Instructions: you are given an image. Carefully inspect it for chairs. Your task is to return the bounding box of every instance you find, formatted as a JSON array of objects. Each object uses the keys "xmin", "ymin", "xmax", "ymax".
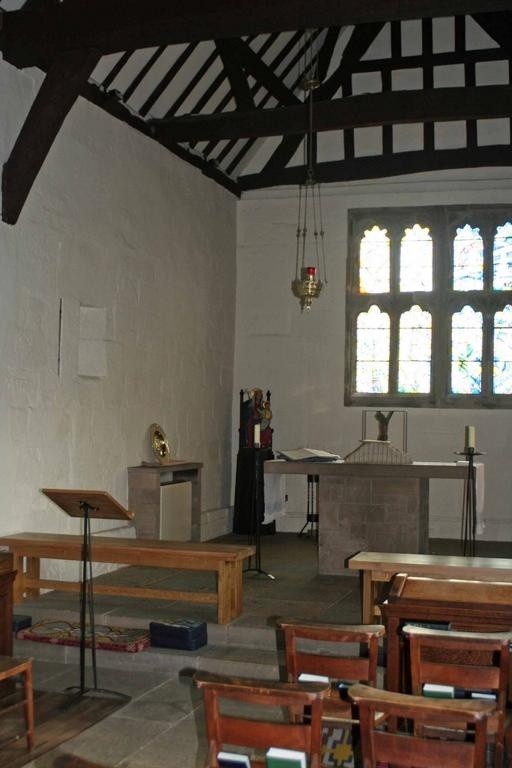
[
  {"xmin": 1, "ymin": 653, "xmax": 37, "ymax": 754},
  {"xmin": 345, "ymin": 682, "xmax": 498, "ymax": 768},
  {"xmin": 192, "ymin": 668, "xmax": 330, "ymax": 768},
  {"xmin": 265, "ymin": 615, "xmax": 386, "ymax": 730},
  {"xmin": 401, "ymin": 625, "xmax": 511, "ymax": 768}
]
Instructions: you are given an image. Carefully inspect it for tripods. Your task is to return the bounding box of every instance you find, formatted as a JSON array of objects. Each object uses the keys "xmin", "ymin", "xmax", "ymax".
[
  {"xmin": 62, "ymin": 518, "xmax": 131, "ymax": 699},
  {"xmin": 242, "ymin": 466, "xmax": 275, "ymax": 579}
]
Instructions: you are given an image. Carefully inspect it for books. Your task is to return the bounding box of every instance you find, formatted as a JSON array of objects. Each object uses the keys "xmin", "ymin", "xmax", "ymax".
[
  {"xmin": 337, "ymin": 682, "xmax": 352, "ymax": 701},
  {"xmin": 265, "ymin": 746, "xmax": 307, "ymax": 767},
  {"xmin": 295, "ymin": 673, "xmax": 330, "ymax": 685},
  {"xmin": 216, "ymin": 751, "xmax": 251, "ymax": 767},
  {"xmin": 470, "ymin": 692, "xmax": 497, "ymax": 700},
  {"xmin": 422, "ymin": 682, "xmax": 455, "ymax": 699}
]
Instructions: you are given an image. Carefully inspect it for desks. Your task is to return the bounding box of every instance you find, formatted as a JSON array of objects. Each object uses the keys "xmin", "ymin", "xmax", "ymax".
[{"xmin": 265, "ymin": 459, "xmax": 484, "ymax": 578}]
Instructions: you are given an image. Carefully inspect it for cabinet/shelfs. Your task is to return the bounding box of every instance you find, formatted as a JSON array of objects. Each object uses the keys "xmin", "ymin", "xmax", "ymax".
[
  {"xmin": 127, "ymin": 461, "xmax": 204, "ymax": 545},
  {"xmin": 376, "ymin": 572, "xmax": 510, "ymax": 732},
  {"xmin": 0, "ymin": 550, "xmax": 18, "ymax": 699}
]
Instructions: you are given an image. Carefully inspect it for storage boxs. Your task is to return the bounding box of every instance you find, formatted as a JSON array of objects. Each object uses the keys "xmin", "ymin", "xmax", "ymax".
[{"xmin": 148, "ymin": 615, "xmax": 208, "ymax": 652}]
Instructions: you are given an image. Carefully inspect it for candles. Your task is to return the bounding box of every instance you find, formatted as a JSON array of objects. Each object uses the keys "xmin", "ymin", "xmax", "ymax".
[
  {"xmin": 253, "ymin": 425, "xmax": 261, "ymax": 444},
  {"xmin": 304, "ymin": 266, "xmax": 317, "ymax": 280},
  {"xmin": 463, "ymin": 424, "xmax": 477, "ymax": 450}
]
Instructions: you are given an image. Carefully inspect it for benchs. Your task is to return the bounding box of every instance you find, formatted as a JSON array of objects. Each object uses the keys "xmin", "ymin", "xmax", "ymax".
[
  {"xmin": 348, "ymin": 550, "xmax": 511, "ymax": 625},
  {"xmin": 1, "ymin": 531, "xmax": 256, "ymax": 625}
]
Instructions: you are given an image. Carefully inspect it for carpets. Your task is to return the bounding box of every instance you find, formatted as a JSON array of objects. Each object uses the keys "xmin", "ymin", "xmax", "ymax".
[{"xmin": 0, "ymin": 687, "xmax": 126, "ymax": 768}]
